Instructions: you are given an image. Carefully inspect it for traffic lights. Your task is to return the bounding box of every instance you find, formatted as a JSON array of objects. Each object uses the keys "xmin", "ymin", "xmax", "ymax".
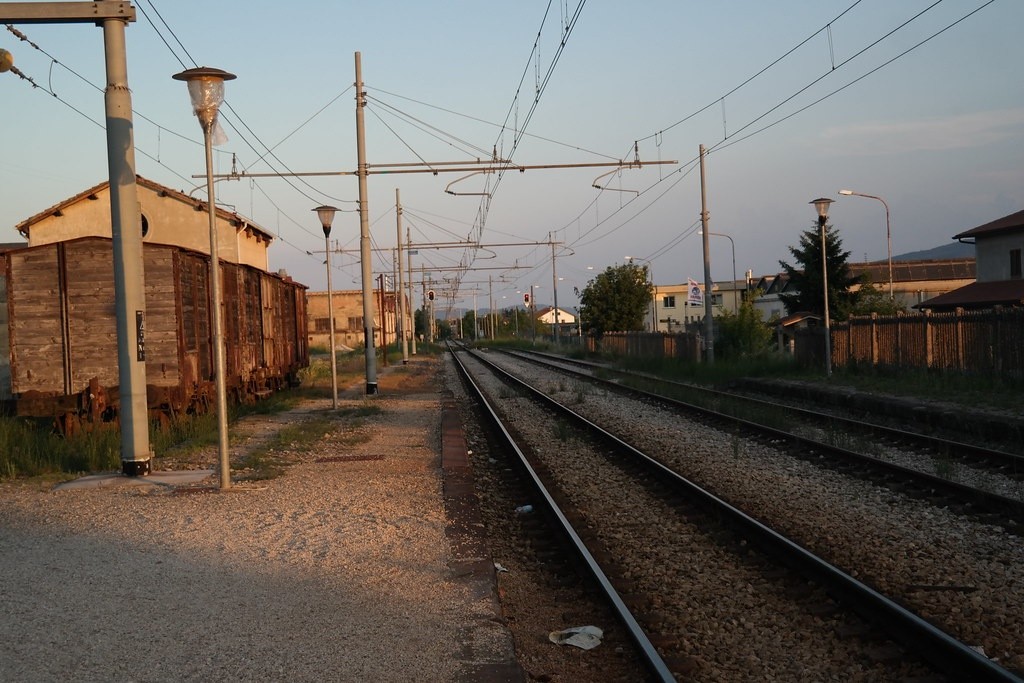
[
  {"xmin": 525, "ymin": 294, "xmax": 529, "ymax": 303},
  {"xmin": 428, "ymin": 291, "xmax": 434, "ymax": 301}
]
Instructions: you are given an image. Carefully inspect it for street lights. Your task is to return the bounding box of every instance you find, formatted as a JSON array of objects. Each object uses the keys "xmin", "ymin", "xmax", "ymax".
[
  {"xmin": 536, "ymin": 286, "xmax": 554, "ymax": 339},
  {"xmin": 697, "ymin": 230, "xmax": 738, "ymax": 359},
  {"xmin": 502, "ymin": 296, "xmax": 519, "ymax": 342},
  {"xmin": 559, "ymin": 278, "xmax": 582, "ymax": 345},
  {"xmin": 516, "ymin": 291, "xmax": 533, "ymax": 340},
  {"xmin": 839, "ymin": 191, "xmax": 894, "ymax": 311},
  {"xmin": 810, "ymin": 196, "xmax": 835, "ymax": 375},
  {"xmin": 587, "ymin": 267, "xmax": 607, "ymax": 288},
  {"xmin": 311, "ymin": 207, "xmax": 343, "ymax": 408},
  {"xmin": 175, "ymin": 67, "xmax": 238, "ymax": 487},
  {"xmin": 624, "ymin": 256, "xmax": 655, "ymax": 332}
]
